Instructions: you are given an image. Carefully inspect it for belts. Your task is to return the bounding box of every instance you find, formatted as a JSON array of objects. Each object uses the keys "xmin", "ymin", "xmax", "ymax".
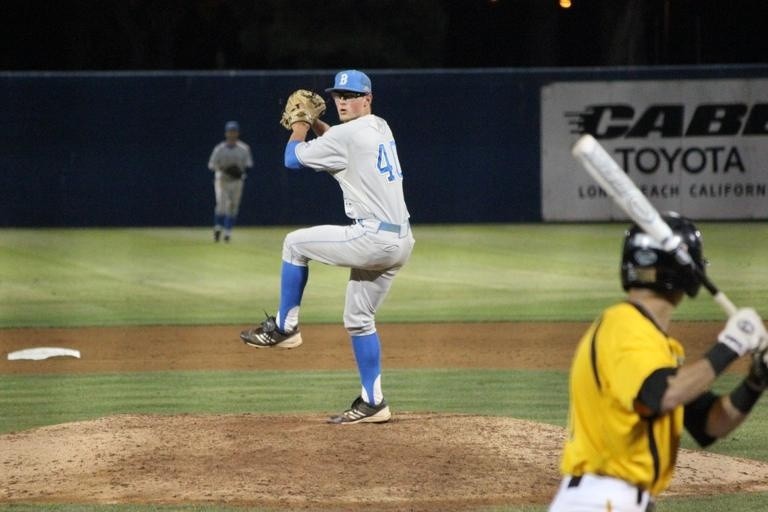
[
  {"xmin": 569, "ymin": 474, "xmax": 656, "ymax": 512},
  {"xmin": 379, "ymin": 222, "xmax": 400, "ymax": 234}
]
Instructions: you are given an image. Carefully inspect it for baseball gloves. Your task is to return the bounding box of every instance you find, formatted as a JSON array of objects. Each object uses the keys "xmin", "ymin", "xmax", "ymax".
[
  {"xmin": 224, "ymin": 165, "xmax": 243, "ymax": 180},
  {"xmin": 282, "ymin": 89, "xmax": 329, "ymax": 129}
]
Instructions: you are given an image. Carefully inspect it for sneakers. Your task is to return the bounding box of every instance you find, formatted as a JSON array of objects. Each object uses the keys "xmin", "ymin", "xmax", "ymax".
[
  {"xmin": 240, "ymin": 315, "xmax": 303, "ymax": 347},
  {"xmin": 327, "ymin": 396, "xmax": 391, "ymax": 425},
  {"xmin": 212, "ymin": 232, "xmax": 230, "ymax": 241}
]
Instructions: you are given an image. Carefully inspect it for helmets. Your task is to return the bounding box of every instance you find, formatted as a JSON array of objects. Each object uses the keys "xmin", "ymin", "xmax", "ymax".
[{"xmin": 620, "ymin": 211, "xmax": 703, "ymax": 303}]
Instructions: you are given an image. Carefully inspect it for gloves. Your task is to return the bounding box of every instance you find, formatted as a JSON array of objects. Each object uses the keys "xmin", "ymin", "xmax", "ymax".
[{"xmin": 717, "ymin": 308, "xmax": 767, "ymax": 388}]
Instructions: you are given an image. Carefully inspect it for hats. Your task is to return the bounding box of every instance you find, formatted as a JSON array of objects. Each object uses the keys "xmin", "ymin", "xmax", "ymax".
[
  {"xmin": 326, "ymin": 70, "xmax": 371, "ymax": 92},
  {"xmin": 227, "ymin": 122, "xmax": 238, "ymax": 130}
]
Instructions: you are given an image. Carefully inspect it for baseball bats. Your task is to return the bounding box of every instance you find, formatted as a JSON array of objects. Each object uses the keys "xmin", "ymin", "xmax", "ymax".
[{"xmin": 571, "ymin": 133, "xmax": 762, "ymax": 347}]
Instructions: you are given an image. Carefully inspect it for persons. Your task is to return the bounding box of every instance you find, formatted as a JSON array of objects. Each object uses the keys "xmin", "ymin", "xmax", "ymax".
[
  {"xmin": 240, "ymin": 70, "xmax": 417, "ymax": 424},
  {"xmin": 547, "ymin": 202, "xmax": 768, "ymax": 512},
  {"xmin": 207, "ymin": 121, "xmax": 255, "ymax": 243}
]
